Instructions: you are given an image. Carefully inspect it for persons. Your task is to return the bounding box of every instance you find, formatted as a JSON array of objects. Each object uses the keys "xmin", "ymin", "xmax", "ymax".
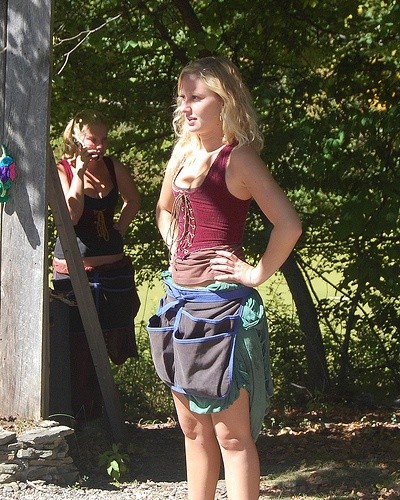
[
  {"xmin": 147, "ymin": 57, "xmax": 302, "ymax": 500},
  {"xmin": 53, "ymin": 109, "xmax": 142, "ymax": 421}
]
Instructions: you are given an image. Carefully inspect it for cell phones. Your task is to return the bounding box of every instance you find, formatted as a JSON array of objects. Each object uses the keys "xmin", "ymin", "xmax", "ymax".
[{"xmin": 71, "ymin": 134, "xmax": 98, "ymax": 161}]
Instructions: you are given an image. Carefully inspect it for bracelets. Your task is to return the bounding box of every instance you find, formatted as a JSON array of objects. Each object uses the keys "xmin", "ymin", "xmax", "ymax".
[{"xmin": 250, "ymin": 267, "xmax": 256, "ymax": 286}]
